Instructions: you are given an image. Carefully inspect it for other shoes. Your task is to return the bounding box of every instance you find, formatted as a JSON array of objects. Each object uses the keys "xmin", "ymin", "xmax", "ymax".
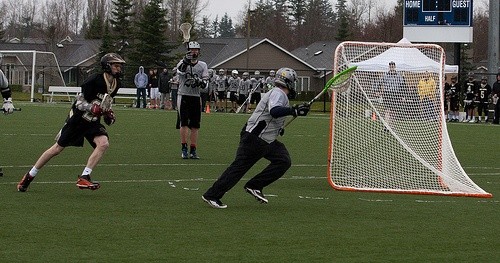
[
  {"xmin": 449, "ymin": 118, "xmax": 489, "ymax": 123},
  {"xmin": 189, "ymin": 151, "xmax": 200, "ymax": 159},
  {"xmin": 181, "ymin": 149, "xmax": 189, "ymax": 158}
]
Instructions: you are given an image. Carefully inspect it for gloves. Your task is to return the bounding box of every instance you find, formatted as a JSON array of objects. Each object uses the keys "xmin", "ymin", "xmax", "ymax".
[
  {"xmin": 104, "ymin": 111, "xmax": 116, "ymax": 126},
  {"xmin": 2, "ymin": 97, "xmax": 15, "ymax": 115},
  {"xmin": 182, "ymin": 50, "xmax": 197, "ymax": 64},
  {"xmin": 190, "ymin": 76, "xmax": 203, "ymax": 87},
  {"xmin": 291, "ymin": 102, "xmax": 310, "ymax": 118},
  {"xmin": 89, "ymin": 102, "xmax": 102, "ymax": 116}
]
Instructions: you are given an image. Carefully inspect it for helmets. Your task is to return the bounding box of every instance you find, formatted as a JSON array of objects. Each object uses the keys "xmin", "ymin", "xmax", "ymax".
[
  {"xmin": 274, "ymin": 67, "xmax": 299, "ymax": 97},
  {"xmin": 101, "ymin": 53, "xmax": 126, "ymax": 81},
  {"xmin": 242, "ymin": 72, "xmax": 249, "ymax": 79},
  {"xmin": 467, "ymin": 72, "xmax": 474, "ymax": 79},
  {"xmin": 232, "ymin": 70, "xmax": 238, "ymax": 79},
  {"xmin": 270, "ymin": 70, "xmax": 276, "ymax": 76},
  {"xmin": 219, "ymin": 69, "xmax": 224, "ymax": 74},
  {"xmin": 187, "ymin": 41, "xmax": 200, "ymax": 49},
  {"xmin": 451, "ymin": 75, "xmax": 457, "ymax": 81}
]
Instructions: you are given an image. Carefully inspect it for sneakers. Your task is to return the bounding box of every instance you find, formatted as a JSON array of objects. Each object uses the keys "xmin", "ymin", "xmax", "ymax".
[
  {"xmin": 16, "ymin": 171, "xmax": 36, "ymax": 192},
  {"xmin": 201, "ymin": 195, "xmax": 228, "ymax": 209},
  {"xmin": 243, "ymin": 185, "xmax": 268, "ymax": 205},
  {"xmin": 75, "ymin": 174, "xmax": 101, "ymax": 191}
]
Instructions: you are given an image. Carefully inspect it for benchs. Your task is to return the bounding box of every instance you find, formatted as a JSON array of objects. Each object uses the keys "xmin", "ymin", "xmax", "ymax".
[{"xmin": 42, "ymin": 86, "xmax": 159, "ymax": 108}]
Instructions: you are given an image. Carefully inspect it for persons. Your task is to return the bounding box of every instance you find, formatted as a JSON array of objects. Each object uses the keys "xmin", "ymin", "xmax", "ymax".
[
  {"xmin": 134, "ymin": 65, "xmax": 276, "ymax": 129},
  {"xmin": 409, "ymin": 70, "xmax": 500, "ymax": 123},
  {"xmin": 177, "ymin": 41, "xmax": 209, "ymax": 159},
  {"xmin": 376, "ymin": 61, "xmax": 405, "ymax": 132},
  {"xmin": 17, "ymin": 52, "xmax": 126, "ymax": 192},
  {"xmin": 201, "ymin": 67, "xmax": 310, "ymax": 209},
  {"xmin": 0, "ymin": 69, "xmax": 14, "ymax": 176}
]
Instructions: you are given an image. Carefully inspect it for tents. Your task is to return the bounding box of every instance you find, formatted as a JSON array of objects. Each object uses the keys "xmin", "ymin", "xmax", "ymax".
[{"xmin": 346, "ymin": 36, "xmax": 458, "ymax": 117}]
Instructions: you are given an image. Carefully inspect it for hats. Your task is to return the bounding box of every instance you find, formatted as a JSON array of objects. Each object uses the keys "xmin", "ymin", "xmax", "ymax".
[
  {"xmin": 481, "ymin": 76, "xmax": 488, "ymax": 80},
  {"xmin": 254, "ymin": 71, "xmax": 260, "ymax": 75}
]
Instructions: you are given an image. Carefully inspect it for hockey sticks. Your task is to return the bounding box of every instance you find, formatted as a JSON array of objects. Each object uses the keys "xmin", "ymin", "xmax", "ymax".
[
  {"xmin": 99, "ymin": 93, "xmax": 113, "ymax": 118},
  {"xmin": 0, "ymin": 108, "xmax": 21, "ymax": 111},
  {"xmin": 281, "ymin": 65, "xmax": 358, "ymax": 130},
  {"xmin": 236, "ymin": 78, "xmax": 264, "ymax": 114},
  {"xmin": 180, "ymin": 22, "xmax": 193, "ymax": 77}
]
